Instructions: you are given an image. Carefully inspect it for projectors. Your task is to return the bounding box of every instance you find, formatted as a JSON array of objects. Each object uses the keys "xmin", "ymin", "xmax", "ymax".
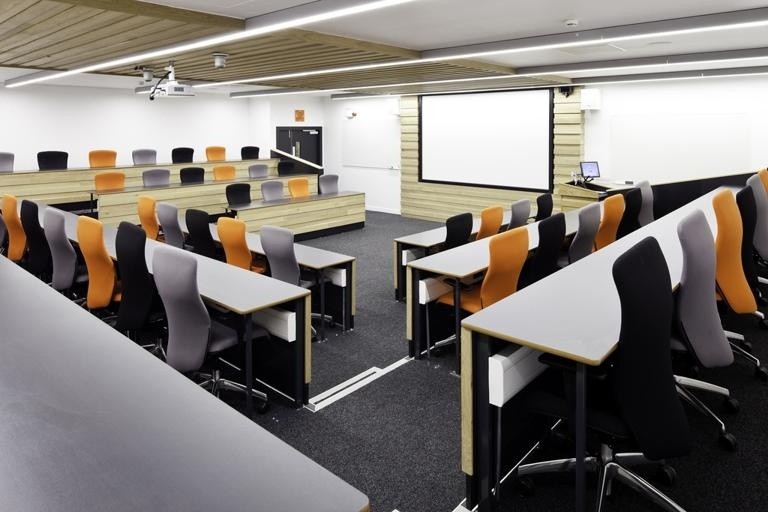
[{"xmin": 134, "ymin": 81, "xmax": 195, "ymax": 101}]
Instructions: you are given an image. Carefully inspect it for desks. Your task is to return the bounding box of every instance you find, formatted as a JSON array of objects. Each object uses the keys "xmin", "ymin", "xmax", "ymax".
[
  {"xmin": 0, "ymin": 256, "xmax": 370, "ymax": 512},
  {"xmin": 394, "ymin": 205, "xmax": 539, "ymax": 301}
]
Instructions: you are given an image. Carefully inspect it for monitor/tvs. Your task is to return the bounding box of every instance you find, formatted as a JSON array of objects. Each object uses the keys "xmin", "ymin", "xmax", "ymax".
[{"xmin": 580, "ymin": 161, "xmax": 601, "ymax": 178}]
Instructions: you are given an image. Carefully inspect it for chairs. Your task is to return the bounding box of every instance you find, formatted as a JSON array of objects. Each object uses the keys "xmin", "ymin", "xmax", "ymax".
[
  {"xmin": 89, "ymin": 149, "xmax": 117, "ymax": 168},
  {"xmin": 37, "ymin": 151, "xmax": 68, "ymax": 170},
  {"xmin": 172, "ymin": 148, "xmax": 194, "ymax": 163},
  {"xmin": 0, "ymin": 152, "xmax": 14, "ymax": 172},
  {"xmin": 241, "ymin": 146, "xmax": 259, "ymax": 160},
  {"xmin": 206, "ymin": 146, "xmax": 226, "ymax": 161},
  {"xmin": 132, "ymin": 149, "xmax": 157, "ymax": 165}
]
